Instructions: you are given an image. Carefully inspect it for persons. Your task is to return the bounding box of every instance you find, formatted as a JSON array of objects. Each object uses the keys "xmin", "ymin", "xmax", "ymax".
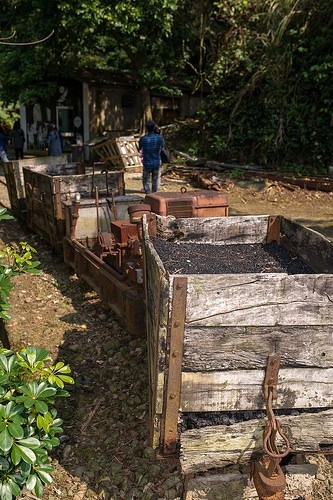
[
  {"xmin": 44, "ymin": 124, "xmax": 61, "ymax": 156},
  {"xmin": 136, "ymin": 120, "xmax": 165, "ymax": 194},
  {"xmin": 10, "ymin": 121, "xmax": 26, "ymax": 161},
  {"xmin": 0, "ymin": 120, "xmax": 11, "ymax": 160}
]
